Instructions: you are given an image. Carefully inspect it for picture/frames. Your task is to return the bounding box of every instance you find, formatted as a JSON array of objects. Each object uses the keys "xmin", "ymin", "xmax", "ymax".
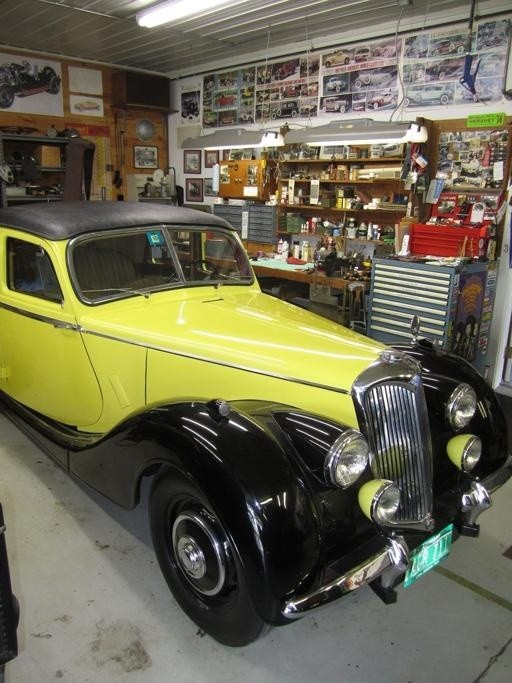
[
  {"xmin": 183, "ymin": 148, "xmax": 254, "ymax": 202},
  {"xmin": 133, "ymin": 144, "xmax": 159, "ymax": 170}
]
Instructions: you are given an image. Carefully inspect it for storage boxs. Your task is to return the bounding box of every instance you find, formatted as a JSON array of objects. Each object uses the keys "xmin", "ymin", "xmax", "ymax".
[{"xmin": 31, "ymin": 145, "xmax": 62, "ymax": 170}]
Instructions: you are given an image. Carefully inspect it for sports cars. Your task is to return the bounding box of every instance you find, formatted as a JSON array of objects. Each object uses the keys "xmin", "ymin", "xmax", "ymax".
[{"xmin": 1, "ymin": 202, "xmax": 510, "ymax": 648}]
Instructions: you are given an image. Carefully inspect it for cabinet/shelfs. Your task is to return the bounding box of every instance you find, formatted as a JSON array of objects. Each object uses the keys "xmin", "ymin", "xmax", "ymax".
[
  {"xmin": 1, "ymin": 133, "xmax": 86, "ymax": 209},
  {"xmin": 276, "ymin": 158, "xmax": 406, "ymax": 246}
]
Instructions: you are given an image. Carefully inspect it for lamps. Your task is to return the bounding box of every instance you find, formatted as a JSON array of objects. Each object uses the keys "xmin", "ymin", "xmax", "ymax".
[
  {"xmin": 134, "ymin": 1, "xmax": 227, "ymax": 29},
  {"xmin": 179, "ymin": 4, "xmax": 428, "ymax": 150}
]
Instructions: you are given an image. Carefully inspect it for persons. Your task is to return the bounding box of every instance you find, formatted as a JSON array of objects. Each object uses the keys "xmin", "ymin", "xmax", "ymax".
[{"xmin": 453, "ymin": 314, "xmax": 479, "ymax": 361}]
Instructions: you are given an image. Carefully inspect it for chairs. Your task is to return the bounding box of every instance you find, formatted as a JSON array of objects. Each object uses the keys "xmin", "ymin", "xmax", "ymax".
[{"xmin": 29, "ymin": 242, "xmax": 162, "ymax": 306}]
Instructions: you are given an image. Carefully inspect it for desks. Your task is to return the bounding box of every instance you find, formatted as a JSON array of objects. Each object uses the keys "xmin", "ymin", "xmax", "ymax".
[{"xmin": 172, "ymin": 254, "xmax": 368, "ymax": 320}]
